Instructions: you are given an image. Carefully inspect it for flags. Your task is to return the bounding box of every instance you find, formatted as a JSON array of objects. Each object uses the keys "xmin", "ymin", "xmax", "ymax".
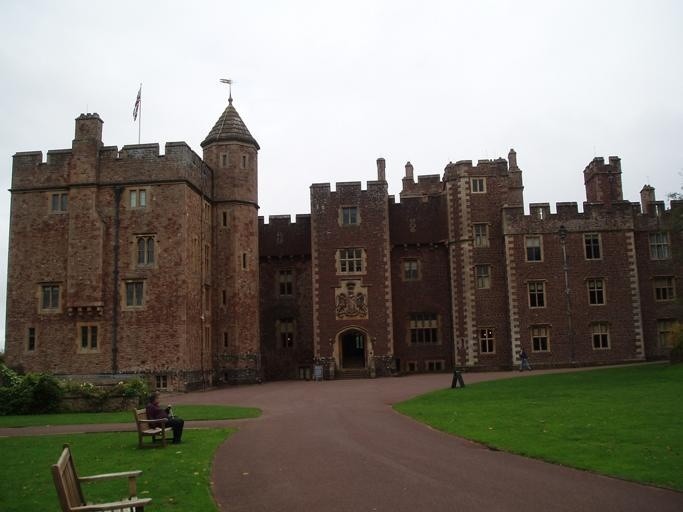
[{"xmin": 132, "ymin": 89, "xmax": 141, "ymax": 121}]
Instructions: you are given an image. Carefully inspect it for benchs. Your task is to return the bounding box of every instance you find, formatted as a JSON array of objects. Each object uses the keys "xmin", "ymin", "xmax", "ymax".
[
  {"xmin": 133, "ymin": 407, "xmax": 175, "ymax": 448},
  {"xmin": 52, "ymin": 444, "xmax": 153, "ymax": 511}
]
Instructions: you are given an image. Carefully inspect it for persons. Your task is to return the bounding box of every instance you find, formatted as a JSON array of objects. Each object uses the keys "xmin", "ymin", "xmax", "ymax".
[
  {"xmin": 518, "ymin": 348, "xmax": 532, "ymax": 371},
  {"xmin": 146, "ymin": 393, "xmax": 184, "ymax": 444}
]
